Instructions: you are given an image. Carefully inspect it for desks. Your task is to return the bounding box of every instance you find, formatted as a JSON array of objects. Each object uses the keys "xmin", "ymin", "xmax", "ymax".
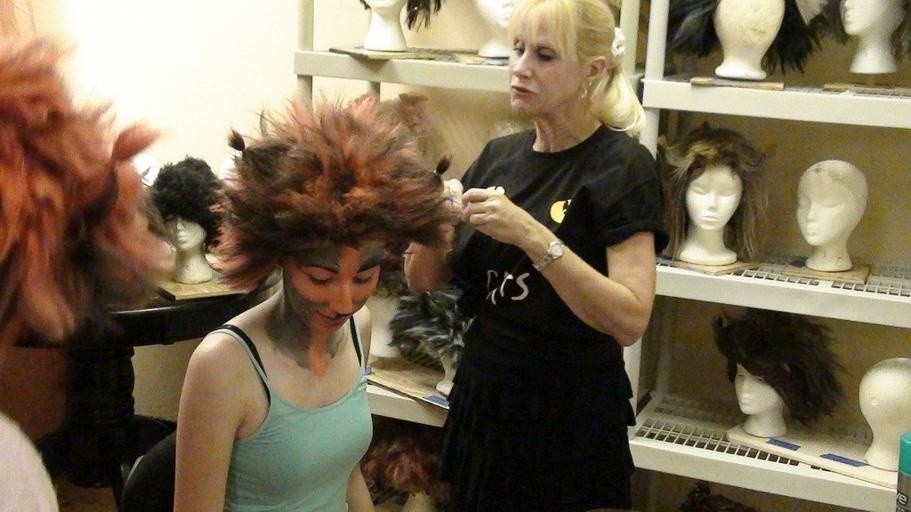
[{"xmin": 8, "ymin": 268, "xmax": 268, "ymax": 489}]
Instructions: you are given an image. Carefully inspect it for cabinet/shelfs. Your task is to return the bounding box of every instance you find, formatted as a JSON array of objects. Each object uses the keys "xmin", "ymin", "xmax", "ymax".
[
  {"xmin": 293, "ymin": 1, "xmax": 641, "ymax": 428},
  {"xmin": 627, "ymin": 1, "xmax": 911, "ymax": 512}
]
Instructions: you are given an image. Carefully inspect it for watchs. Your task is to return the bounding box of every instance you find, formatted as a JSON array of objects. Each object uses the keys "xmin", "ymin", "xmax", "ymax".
[{"xmin": 531, "ymin": 239, "xmax": 566, "ymax": 273}]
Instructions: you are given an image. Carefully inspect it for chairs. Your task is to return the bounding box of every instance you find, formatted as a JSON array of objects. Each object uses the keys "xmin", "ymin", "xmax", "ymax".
[{"xmin": 121, "ymin": 432, "xmax": 177, "ymax": 511}]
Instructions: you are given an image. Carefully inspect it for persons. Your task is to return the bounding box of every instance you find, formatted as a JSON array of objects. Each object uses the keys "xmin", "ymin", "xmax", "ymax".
[
  {"xmin": 665, "ymin": 0, "xmax": 824, "ymax": 82},
  {"xmin": 711, "ymin": 306, "xmax": 845, "ymax": 437},
  {"xmin": 405, "ymin": 2, "xmax": 670, "ymax": 511},
  {"xmin": 795, "ymin": 161, "xmax": 873, "ymax": 272},
  {"xmin": 858, "ymin": 355, "xmax": 910, "ymax": 468},
  {"xmin": 810, "ymin": 1, "xmax": 910, "ymax": 78},
  {"xmin": 1, "ymin": 0, "xmax": 168, "ymax": 509},
  {"xmin": 170, "ymin": 95, "xmax": 463, "ymax": 510},
  {"xmin": 359, "ymin": 0, "xmax": 443, "ymax": 56},
  {"xmin": 147, "ymin": 156, "xmax": 230, "ymax": 285},
  {"xmin": 469, "ymin": 0, "xmax": 527, "ymax": 61},
  {"xmin": 658, "ymin": 116, "xmax": 773, "ymax": 266}
]
